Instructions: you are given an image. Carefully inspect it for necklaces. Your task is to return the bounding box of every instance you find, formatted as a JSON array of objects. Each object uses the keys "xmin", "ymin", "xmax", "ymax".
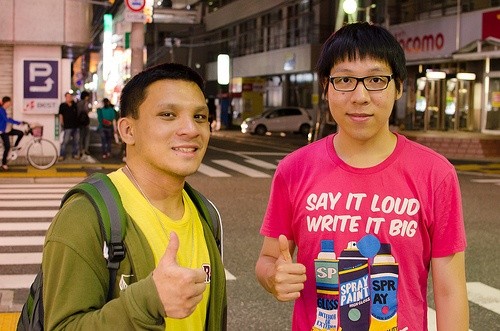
[{"xmin": 126, "ymin": 164, "xmax": 195, "ymax": 268}]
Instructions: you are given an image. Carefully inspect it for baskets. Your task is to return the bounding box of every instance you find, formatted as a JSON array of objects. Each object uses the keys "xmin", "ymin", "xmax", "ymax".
[{"xmin": 32, "ymin": 126, "xmax": 43, "ymax": 137}]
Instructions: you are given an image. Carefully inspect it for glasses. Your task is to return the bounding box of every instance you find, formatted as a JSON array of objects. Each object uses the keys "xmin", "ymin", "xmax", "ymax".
[{"xmin": 328, "ymin": 72, "xmax": 401, "ymax": 92}]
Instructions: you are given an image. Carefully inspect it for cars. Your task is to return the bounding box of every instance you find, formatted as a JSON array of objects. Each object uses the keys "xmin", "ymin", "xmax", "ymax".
[{"xmin": 241, "ymin": 106, "xmax": 313, "ymax": 138}]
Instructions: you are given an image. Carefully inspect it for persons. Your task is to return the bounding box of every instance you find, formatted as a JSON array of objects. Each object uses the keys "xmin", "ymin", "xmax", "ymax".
[
  {"xmin": 0, "ymin": 96, "xmax": 27, "ymax": 171},
  {"xmin": 43, "ymin": 63, "xmax": 227, "ymax": 331},
  {"xmin": 255, "ymin": 23, "xmax": 469, "ymax": 331},
  {"xmin": 57, "ymin": 91, "xmax": 127, "ymax": 162}
]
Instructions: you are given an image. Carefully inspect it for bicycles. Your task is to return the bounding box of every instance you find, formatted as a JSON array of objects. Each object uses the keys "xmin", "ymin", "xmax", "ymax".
[{"xmin": 0, "ymin": 122, "xmax": 58, "ymax": 171}]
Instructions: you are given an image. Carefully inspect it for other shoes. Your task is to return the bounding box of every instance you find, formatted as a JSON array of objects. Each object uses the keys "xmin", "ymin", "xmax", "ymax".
[
  {"xmin": 2, "ymin": 164, "xmax": 8, "ymax": 169},
  {"xmin": 16, "ymin": 145, "xmax": 21, "ymax": 148},
  {"xmin": 57, "ymin": 155, "xmax": 63, "ymax": 161},
  {"xmin": 72, "ymin": 154, "xmax": 78, "ymax": 159}
]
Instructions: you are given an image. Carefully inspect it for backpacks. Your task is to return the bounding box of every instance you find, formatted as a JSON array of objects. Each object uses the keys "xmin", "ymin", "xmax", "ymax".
[{"xmin": 16, "ymin": 172, "xmax": 222, "ymax": 331}]
